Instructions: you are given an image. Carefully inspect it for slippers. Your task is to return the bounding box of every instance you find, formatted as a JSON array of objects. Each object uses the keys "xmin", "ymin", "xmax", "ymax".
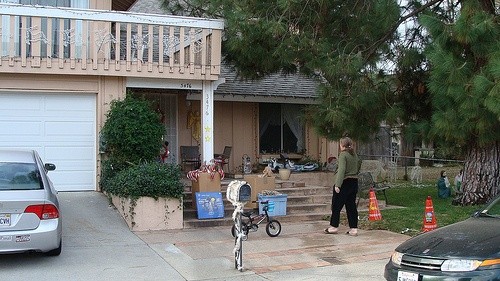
[
  {"xmin": 323, "ymin": 228, "xmax": 338, "ymax": 235},
  {"xmin": 346, "ymin": 229, "xmax": 357, "ymax": 237}
]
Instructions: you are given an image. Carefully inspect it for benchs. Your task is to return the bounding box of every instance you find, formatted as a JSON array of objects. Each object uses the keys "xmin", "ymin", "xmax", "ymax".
[{"xmin": 356, "ymin": 172, "xmax": 390, "ymax": 207}]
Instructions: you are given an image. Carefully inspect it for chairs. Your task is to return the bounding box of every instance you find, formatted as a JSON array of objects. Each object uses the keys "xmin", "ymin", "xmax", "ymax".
[
  {"xmin": 214, "ymin": 146, "xmax": 232, "ymax": 173},
  {"xmin": 180, "ymin": 146, "xmax": 201, "ymax": 172}
]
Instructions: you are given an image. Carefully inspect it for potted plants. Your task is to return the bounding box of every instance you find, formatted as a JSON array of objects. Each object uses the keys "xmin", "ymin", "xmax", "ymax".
[{"xmin": 276, "ymin": 154, "xmax": 291, "ymax": 180}]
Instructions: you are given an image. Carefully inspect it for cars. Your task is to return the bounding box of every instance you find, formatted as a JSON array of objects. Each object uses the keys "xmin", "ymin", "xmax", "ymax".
[
  {"xmin": 384, "ymin": 193, "xmax": 500, "ymax": 281},
  {"xmin": 0, "ymin": 149, "xmax": 63, "ymax": 256}
]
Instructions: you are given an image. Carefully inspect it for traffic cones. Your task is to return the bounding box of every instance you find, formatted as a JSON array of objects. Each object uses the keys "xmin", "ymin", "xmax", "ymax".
[
  {"xmin": 369, "ymin": 188, "xmax": 381, "ymax": 222},
  {"xmin": 422, "ymin": 196, "xmax": 440, "ymax": 233}
]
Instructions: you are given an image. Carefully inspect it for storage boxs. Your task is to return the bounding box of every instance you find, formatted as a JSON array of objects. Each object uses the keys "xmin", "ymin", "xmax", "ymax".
[
  {"xmin": 257, "ymin": 194, "xmax": 288, "ymax": 216},
  {"xmin": 243, "ymin": 174, "xmax": 276, "ymax": 208},
  {"xmin": 192, "ymin": 172, "xmax": 221, "ymax": 208}
]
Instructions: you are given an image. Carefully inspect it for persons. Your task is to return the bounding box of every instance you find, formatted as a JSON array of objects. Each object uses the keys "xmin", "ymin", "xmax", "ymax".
[
  {"xmin": 438, "ymin": 169, "xmax": 464, "ymax": 200},
  {"xmin": 323, "ymin": 136, "xmax": 362, "ymax": 237}
]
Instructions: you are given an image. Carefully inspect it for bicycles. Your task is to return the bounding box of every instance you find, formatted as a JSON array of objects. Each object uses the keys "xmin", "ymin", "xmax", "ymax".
[{"xmin": 230, "ymin": 199, "xmax": 282, "ymax": 241}]
